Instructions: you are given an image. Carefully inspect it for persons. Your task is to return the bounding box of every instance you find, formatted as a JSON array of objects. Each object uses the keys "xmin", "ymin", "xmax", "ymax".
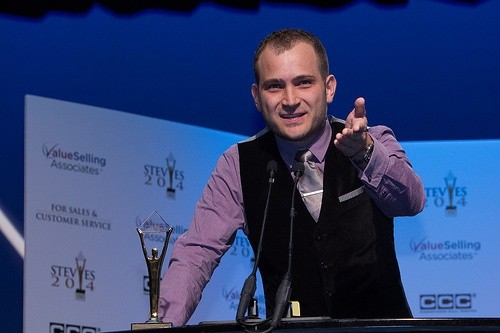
[
  {"xmin": 135, "ymin": 225, "xmax": 174, "ymax": 324},
  {"xmin": 150, "ymin": 28, "xmax": 427, "ymax": 326}
]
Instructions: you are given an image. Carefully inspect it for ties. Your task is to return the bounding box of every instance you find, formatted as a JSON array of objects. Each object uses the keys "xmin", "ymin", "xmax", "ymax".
[{"xmin": 297, "ymin": 149, "xmax": 323, "ymax": 223}]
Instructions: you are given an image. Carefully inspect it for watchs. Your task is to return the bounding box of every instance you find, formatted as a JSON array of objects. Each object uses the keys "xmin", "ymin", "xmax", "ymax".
[{"xmin": 350, "ymin": 143, "xmax": 376, "ymax": 163}]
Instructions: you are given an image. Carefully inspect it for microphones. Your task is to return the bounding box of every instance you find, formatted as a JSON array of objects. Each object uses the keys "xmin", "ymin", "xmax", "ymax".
[
  {"xmin": 271, "ymin": 161, "xmax": 305, "ymax": 328},
  {"xmin": 236, "ymin": 161, "xmax": 278, "ymax": 322}
]
